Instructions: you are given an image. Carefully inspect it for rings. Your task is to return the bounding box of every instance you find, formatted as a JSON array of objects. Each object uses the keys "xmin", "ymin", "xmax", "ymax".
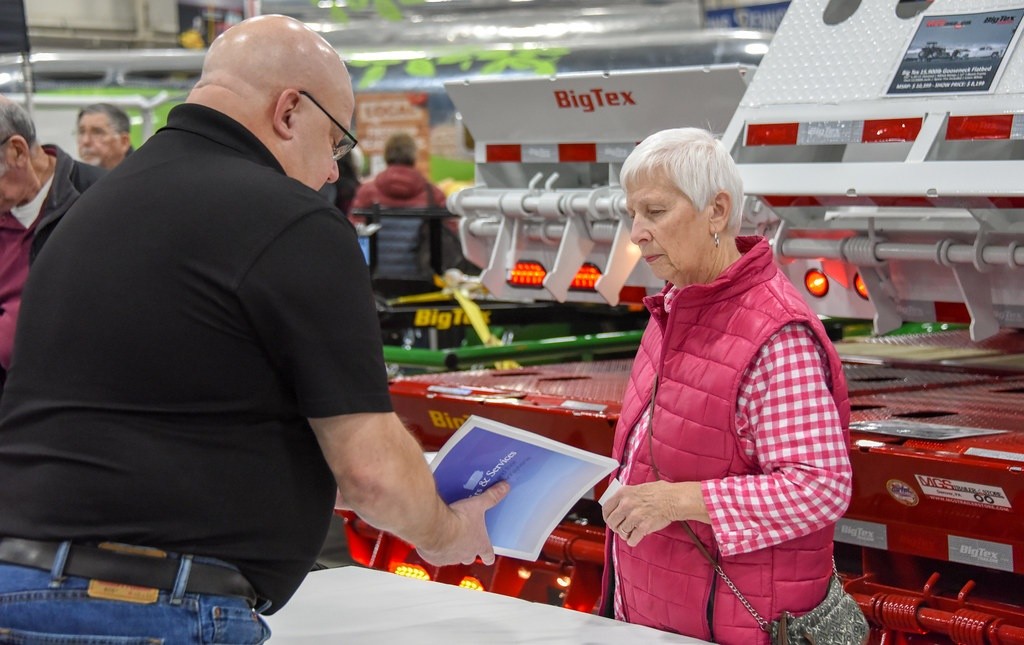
[{"xmin": 620, "ymin": 526, "xmax": 629, "ymax": 535}]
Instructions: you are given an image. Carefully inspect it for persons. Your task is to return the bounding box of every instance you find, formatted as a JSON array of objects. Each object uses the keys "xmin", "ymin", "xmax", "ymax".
[
  {"xmin": 598, "ymin": 125, "xmax": 853, "ymax": 644},
  {"xmin": 1, "ymin": 13, "xmax": 510, "ymax": 645}
]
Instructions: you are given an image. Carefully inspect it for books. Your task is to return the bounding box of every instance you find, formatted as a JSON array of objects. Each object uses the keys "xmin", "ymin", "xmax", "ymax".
[{"xmin": 423, "ymin": 414, "xmax": 620, "ymax": 562}]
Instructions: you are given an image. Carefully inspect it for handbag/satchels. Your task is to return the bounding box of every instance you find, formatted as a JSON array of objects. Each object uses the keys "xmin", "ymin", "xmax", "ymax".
[{"xmin": 764, "ymin": 577, "xmax": 870, "ymax": 645}]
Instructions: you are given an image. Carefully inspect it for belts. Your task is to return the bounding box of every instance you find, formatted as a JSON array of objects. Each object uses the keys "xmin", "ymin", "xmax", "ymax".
[{"xmin": 0, "ymin": 536, "xmax": 259, "ymax": 606}]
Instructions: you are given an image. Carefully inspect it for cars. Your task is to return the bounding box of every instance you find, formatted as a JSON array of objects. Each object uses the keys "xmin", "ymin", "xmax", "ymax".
[{"xmin": 949, "ymin": 43, "xmax": 1002, "ymax": 61}]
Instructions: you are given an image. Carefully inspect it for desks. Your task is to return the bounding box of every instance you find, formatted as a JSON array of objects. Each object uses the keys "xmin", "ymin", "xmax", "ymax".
[{"xmin": 253, "ymin": 566, "xmax": 718, "ymax": 645}]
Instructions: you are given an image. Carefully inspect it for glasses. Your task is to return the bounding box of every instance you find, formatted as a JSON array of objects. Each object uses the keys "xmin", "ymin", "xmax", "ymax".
[{"xmin": 298, "ymin": 90, "xmax": 357, "ymax": 161}]
